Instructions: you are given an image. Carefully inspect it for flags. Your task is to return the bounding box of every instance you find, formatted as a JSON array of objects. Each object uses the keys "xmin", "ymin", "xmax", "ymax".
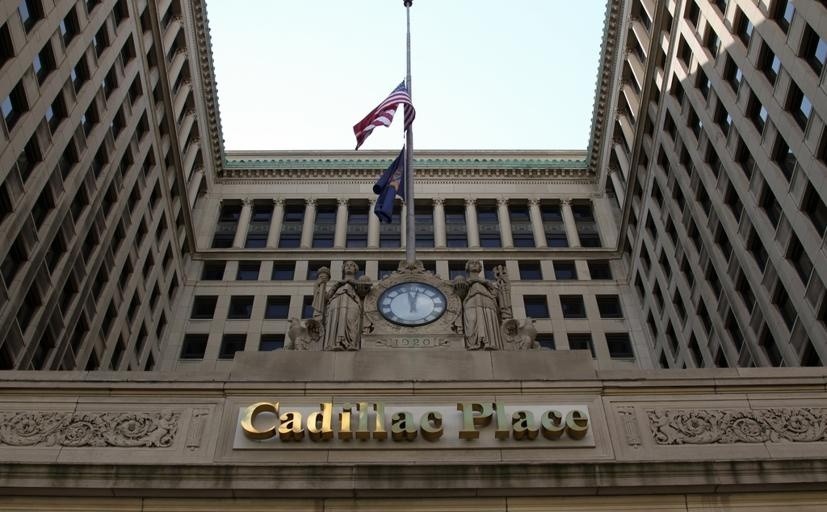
[
  {"xmin": 373, "ymin": 144, "xmax": 405, "ymax": 224},
  {"xmin": 353, "ymin": 81, "xmax": 416, "ymax": 151}
]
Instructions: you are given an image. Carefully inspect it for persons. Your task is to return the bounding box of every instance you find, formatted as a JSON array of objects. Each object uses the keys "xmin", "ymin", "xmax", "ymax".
[
  {"xmin": 312, "ymin": 261, "xmax": 370, "ymax": 351},
  {"xmin": 453, "ymin": 257, "xmax": 507, "ymax": 351}
]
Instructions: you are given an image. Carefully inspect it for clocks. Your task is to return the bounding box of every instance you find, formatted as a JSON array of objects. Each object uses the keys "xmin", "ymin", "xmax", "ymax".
[{"xmin": 376, "ymin": 282, "xmax": 447, "ymax": 326}]
